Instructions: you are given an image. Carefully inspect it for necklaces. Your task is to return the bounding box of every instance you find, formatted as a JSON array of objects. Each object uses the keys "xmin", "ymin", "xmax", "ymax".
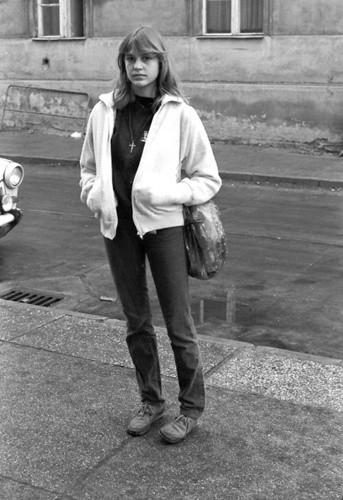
[{"xmin": 124, "ymin": 100, "xmax": 154, "ymax": 152}]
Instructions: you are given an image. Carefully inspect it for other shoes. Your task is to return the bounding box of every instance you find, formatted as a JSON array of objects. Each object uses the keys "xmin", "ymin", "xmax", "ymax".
[
  {"xmin": 128, "ymin": 403, "xmax": 165, "ymax": 435},
  {"xmin": 158, "ymin": 415, "xmax": 204, "ymax": 444}
]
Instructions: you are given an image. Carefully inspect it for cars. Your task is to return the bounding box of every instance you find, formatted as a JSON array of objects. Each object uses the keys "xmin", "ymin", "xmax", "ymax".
[{"xmin": 0, "ymin": 156, "xmax": 25, "ymax": 241}]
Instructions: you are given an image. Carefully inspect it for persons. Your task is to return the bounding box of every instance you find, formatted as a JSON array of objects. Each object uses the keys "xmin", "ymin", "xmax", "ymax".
[{"xmin": 78, "ymin": 27, "xmax": 222, "ymax": 443}]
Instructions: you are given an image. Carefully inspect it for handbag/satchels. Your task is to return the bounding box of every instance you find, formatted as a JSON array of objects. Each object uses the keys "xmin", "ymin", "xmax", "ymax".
[{"xmin": 184, "ymin": 201, "xmax": 228, "ymax": 281}]
